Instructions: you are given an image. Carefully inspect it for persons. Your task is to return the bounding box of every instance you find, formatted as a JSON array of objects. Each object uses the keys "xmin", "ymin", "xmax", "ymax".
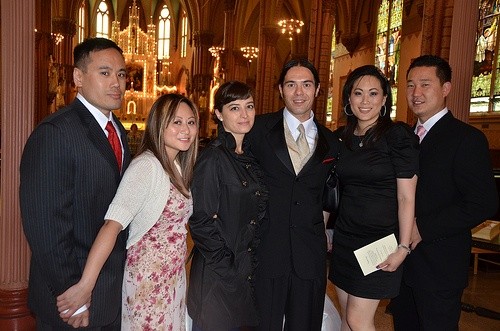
[
  {"xmin": 244, "ymin": 60, "xmax": 340, "ymax": 331},
  {"xmin": 329, "ymin": 65, "xmax": 419, "ymax": 331},
  {"xmin": 56, "ymin": 94, "xmax": 199, "ymax": 331},
  {"xmin": 390, "ymin": 55, "xmax": 494, "ymax": 331},
  {"xmin": 19, "ymin": 38, "xmax": 132, "ymax": 331},
  {"xmin": 187, "ymin": 80, "xmax": 270, "ymax": 331}
]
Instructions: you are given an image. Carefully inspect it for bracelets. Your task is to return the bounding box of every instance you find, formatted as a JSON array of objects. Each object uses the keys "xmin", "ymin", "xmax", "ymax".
[{"xmin": 398, "ymin": 244, "xmax": 411, "ymax": 254}]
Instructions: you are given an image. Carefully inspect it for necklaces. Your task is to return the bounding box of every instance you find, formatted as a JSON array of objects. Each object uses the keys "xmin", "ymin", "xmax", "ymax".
[{"xmin": 359, "ymin": 133, "xmax": 365, "ymax": 147}]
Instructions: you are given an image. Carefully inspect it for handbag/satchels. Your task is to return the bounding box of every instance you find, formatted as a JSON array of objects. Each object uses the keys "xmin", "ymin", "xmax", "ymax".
[{"xmin": 321, "ymin": 164, "xmax": 341, "ymax": 214}]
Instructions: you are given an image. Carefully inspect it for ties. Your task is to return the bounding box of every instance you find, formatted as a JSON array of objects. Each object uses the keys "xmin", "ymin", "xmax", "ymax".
[
  {"xmin": 416, "ymin": 125, "xmax": 426, "ymax": 141},
  {"xmin": 105, "ymin": 120, "xmax": 122, "ymax": 172},
  {"xmin": 296, "ymin": 124, "xmax": 310, "ymax": 161}
]
